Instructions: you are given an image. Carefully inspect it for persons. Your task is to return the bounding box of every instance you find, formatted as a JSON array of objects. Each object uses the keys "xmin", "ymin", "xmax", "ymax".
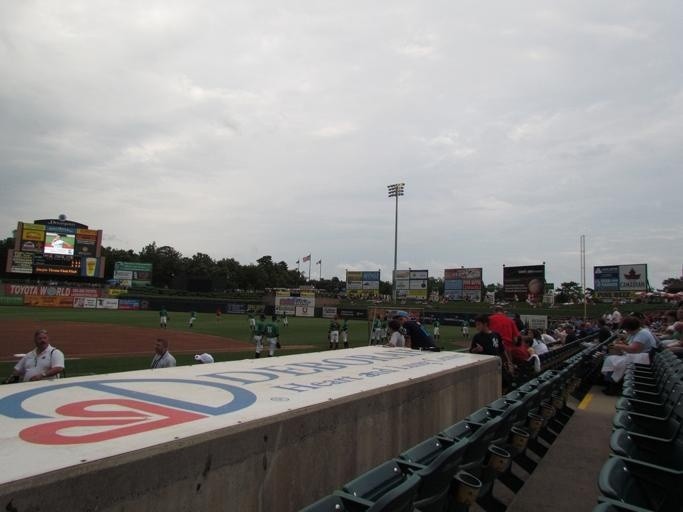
[
  {"xmin": 51, "ymin": 236, "xmax": 70, "ymax": 249},
  {"xmin": 188, "ymin": 308, "xmax": 196, "ymax": 329},
  {"xmin": 2, "ymin": 327, "xmax": 65, "ymax": 383},
  {"xmin": 193, "ymin": 352, "xmax": 214, "ymax": 364},
  {"xmin": 159, "ymin": 306, "xmax": 168, "ymax": 329},
  {"xmin": 215, "ymin": 307, "xmax": 221, "ymax": 325},
  {"xmin": 151, "ymin": 338, "xmax": 175, "ymax": 368}
]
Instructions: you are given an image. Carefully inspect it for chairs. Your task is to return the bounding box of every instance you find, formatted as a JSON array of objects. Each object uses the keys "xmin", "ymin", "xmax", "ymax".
[
  {"xmin": 398, "ymin": 416, "xmax": 504, "ymax": 472},
  {"xmin": 590, "ymin": 494, "xmax": 650, "ymax": 512},
  {"xmin": 609, "ymin": 422, "xmax": 683, "ymax": 472},
  {"xmin": 612, "ymin": 393, "xmax": 682, "ymax": 439},
  {"xmin": 341, "ymin": 436, "xmax": 470, "ymax": 512},
  {"xmin": 615, "ymin": 332, "xmax": 683, "ymax": 417},
  {"xmin": 295, "ymin": 473, "xmax": 421, "ymax": 512},
  {"xmin": 457, "ymin": 331, "xmax": 618, "ymax": 445},
  {"xmin": 598, "ymin": 453, "xmax": 683, "ymax": 512}
]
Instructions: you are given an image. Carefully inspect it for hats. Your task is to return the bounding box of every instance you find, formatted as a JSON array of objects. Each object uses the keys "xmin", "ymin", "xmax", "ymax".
[
  {"xmin": 392, "ymin": 311, "xmax": 409, "ymax": 319},
  {"xmin": 194, "ymin": 353, "xmax": 215, "ymax": 363},
  {"xmin": 260, "ymin": 313, "xmax": 266, "ymax": 319},
  {"xmin": 272, "ymin": 315, "xmax": 277, "ymax": 320}
]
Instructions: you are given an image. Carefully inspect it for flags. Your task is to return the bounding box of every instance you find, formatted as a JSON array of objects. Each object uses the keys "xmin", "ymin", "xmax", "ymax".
[
  {"xmin": 302, "ymin": 255, "xmax": 310, "ymax": 262},
  {"xmin": 316, "ymin": 260, "xmax": 320, "ymax": 264},
  {"xmin": 295, "ymin": 260, "xmax": 299, "ymax": 264}
]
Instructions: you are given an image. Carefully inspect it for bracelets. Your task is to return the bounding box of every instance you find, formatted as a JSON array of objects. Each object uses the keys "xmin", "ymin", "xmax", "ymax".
[{"xmin": 40, "ymin": 372, "xmax": 46, "ymax": 377}]
